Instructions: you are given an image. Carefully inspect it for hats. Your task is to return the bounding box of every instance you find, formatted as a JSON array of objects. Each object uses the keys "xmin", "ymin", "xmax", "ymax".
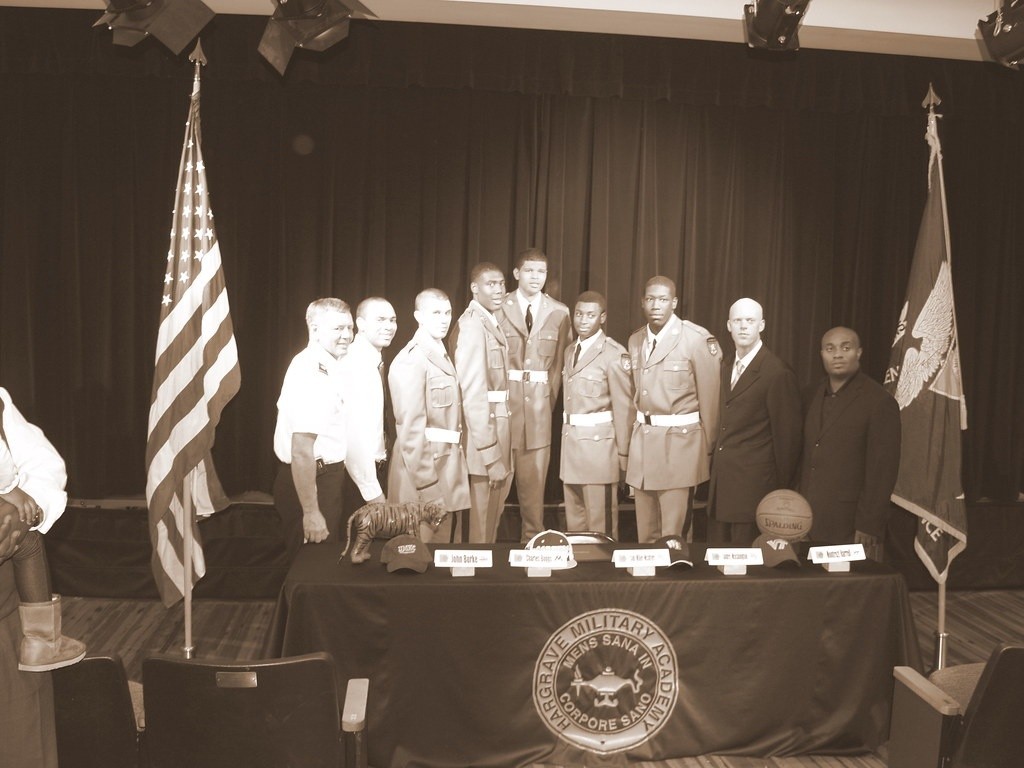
[
  {"xmin": 751, "ymin": 533, "xmax": 801, "ymax": 568},
  {"xmin": 380, "ymin": 535, "xmax": 432, "ymax": 574},
  {"xmin": 652, "ymin": 535, "xmax": 694, "ymax": 568},
  {"xmin": 525, "ymin": 530, "xmax": 578, "ymax": 570}
]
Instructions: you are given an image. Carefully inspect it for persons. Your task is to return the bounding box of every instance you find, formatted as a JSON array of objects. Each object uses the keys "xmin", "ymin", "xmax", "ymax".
[
  {"xmin": 449, "ymin": 262, "xmax": 515, "ymax": 543},
  {"xmin": 706, "ymin": 298, "xmax": 804, "ymax": 545},
  {"xmin": 0, "ymin": 386, "xmax": 87, "ymax": 671},
  {"xmin": 387, "ymin": 287, "xmax": 472, "ymax": 542},
  {"xmin": 558, "ymin": 290, "xmax": 637, "ymax": 541},
  {"xmin": 796, "ymin": 326, "xmax": 902, "ymax": 547},
  {"xmin": 625, "ymin": 276, "xmax": 723, "ymax": 543},
  {"xmin": 273, "ymin": 297, "xmax": 397, "ymax": 561},
  {"xmin": 493, "ymin": 248, "xmax": 574, "ymax": 543}
]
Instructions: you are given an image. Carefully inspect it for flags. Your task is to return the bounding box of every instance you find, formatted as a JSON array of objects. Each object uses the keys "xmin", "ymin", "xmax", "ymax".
[
  {"xmin": 146, "ymin": 88, "xmax": 241, "ymax": 610},
  {"xmin": 883, "ymin": 132, "xmax": 967, "ymax": 584}
]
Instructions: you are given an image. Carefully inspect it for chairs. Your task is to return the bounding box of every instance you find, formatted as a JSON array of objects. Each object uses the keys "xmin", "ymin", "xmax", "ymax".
[
  {"xmin": 878, "ymin": 644, "xmax": 1023, "ymax": 768},
  {"xmin": 48, "ymin": 654, "xmax": 152, "ymax": 768},
  {"xmin": 143, "ymin": 653, "xmax": 370, "ymax": 767}
]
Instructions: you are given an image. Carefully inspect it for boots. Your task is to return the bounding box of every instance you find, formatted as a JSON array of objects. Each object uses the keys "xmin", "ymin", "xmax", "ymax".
[{"xmin": 19, "ymin": 594, "xmax": 86, "ymax": 672}]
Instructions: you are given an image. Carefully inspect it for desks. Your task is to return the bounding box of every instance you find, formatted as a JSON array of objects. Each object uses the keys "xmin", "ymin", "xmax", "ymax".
[
  {"xmin": 42, "ymin": 492, "xmax": 285, "ymax": 603},
  {"xmin": 280, "ymin": 545, "xmax": 924, "ymax": 765},
  {"xmin": 884, "ymin": 493, "xmax": 1024, "ymax": 591}
]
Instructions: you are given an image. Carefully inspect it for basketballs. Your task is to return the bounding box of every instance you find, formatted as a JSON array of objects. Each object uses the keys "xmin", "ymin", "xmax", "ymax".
[{"xmin": 755, "ymin": 488, "xmax": 813, "ymax": 544}]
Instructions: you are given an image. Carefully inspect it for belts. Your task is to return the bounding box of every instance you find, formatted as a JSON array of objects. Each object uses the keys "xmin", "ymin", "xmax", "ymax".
[
  {"xmin": 325, "ymin": 460, "xmax": 344, "ymax": 471},
  {"xmin": 563, "ymin": 411, "xmax": 614, "ymax": 425},
  {"xmin": 376, "ymin": 460, "xmax": 388, "ymax": 470},
  {"xmin": 507, "ymin": 369, "xmax": 548, "ymax": 382},
  {"xmin": 425, "ymin": 427, "xmax": 463, "ymax": 443},
  {"xmin": 637, "ymin": 411, "xmax": 700, "ymax": 426},
  {"xmin": 487, "ymin": 389, "xmax": 509, "ymax": 402}
]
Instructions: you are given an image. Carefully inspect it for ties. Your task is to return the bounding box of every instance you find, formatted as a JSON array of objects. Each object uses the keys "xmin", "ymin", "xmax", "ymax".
[
  {"xmin": 573, "ymin": 344, "xmax": 581, "ymax": 368},
  {"xmin": 731, "ymin": 361, "xmax": 742, "ymax": 391},
  {"xmin": 525, "ymin": 304, "xmax": 533, "ymax": 335},
  {"xmin": 650, "ymin": 338, "xmax": 657, "ymax": 357}
]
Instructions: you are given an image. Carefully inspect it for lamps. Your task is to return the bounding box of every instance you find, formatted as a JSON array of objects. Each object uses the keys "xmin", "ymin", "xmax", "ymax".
[
  {"xmin": 743, "ymin": 0, "xmax": 811, "ymax": 61},
  {"xmin": 91, "ymin": 0, "xmax": 216, "ymax": 56},
  {"xmin": 976, "ymin": 0, "xmax": 1024, "ymax": 71},
  {"xmin": 257, "ymin": 0, "xmax": 379, "ymax": 78}
]
